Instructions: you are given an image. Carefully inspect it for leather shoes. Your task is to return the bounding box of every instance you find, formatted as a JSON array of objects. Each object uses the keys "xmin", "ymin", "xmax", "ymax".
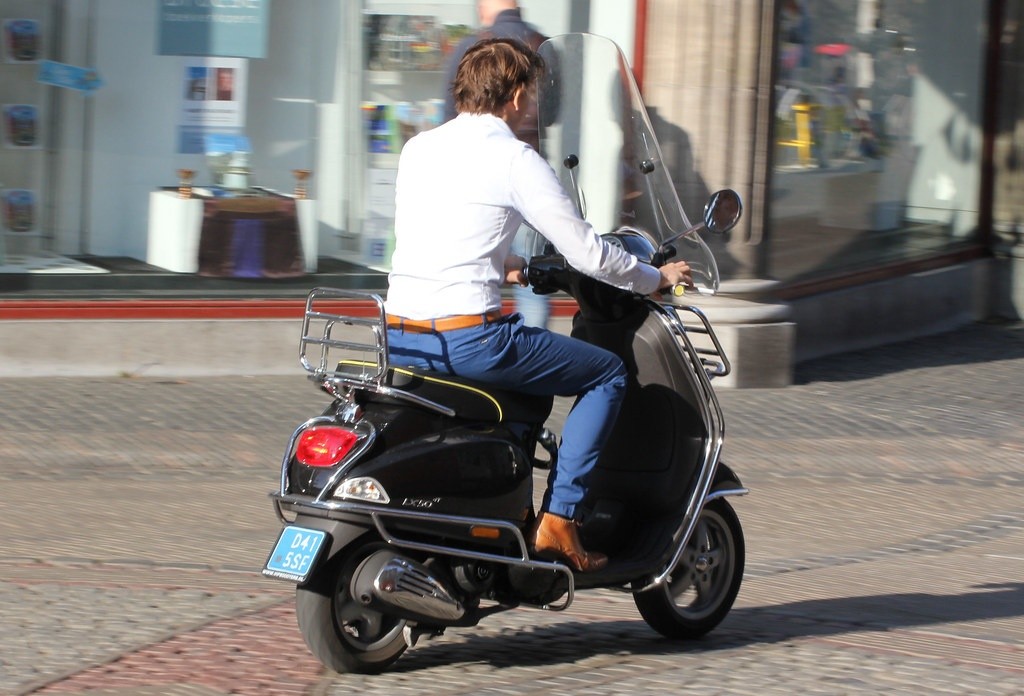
[{"xmin": 526, "ymin": 511, "xmax": 608, "ymax": 573}]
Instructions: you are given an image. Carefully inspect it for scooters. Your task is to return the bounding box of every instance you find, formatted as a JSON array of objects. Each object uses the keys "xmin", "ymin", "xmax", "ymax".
[{"xmin": 252, "ymin": 29, "xmax": 752, "ymax": 678}]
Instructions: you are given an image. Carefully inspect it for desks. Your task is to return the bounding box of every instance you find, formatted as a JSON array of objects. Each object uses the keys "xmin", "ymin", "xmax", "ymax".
[{"xmin": 145, "ymin": 185, "xmax": 321, "ymax": 278}]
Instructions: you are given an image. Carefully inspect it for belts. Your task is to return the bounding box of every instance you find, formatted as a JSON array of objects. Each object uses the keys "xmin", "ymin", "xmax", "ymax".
[{"xmin": 385, "ymin": 310, "xmax": 500, "ymax": 333}]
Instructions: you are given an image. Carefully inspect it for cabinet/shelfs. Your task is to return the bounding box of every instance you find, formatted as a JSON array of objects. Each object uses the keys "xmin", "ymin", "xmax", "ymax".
[{"xmin": 0, "ymin": 58, "xmax": 58, "ymax": 239}]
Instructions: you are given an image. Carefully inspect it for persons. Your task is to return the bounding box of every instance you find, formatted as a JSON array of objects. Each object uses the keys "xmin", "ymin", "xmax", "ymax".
[
  {"xmin": 817, "ymin": 66, "xmax": 846, "ymax": 167},
  {"xmin": 383, "ymin": 37, "xmax": 694, "ymax": 572},
  {"xmin": 442, "ymin": 0, "xmax": 561, "ymax": 330}
]
